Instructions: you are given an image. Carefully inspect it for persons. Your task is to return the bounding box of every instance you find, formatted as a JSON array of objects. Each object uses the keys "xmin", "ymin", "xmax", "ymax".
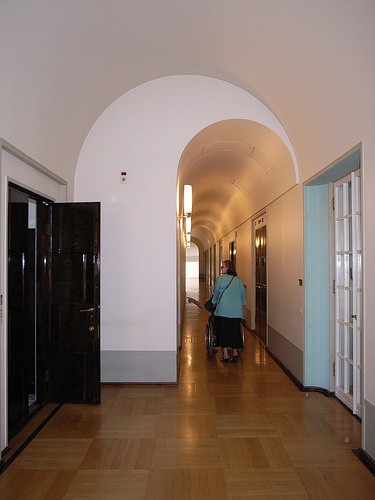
[
  {"xmin": 211, "ymin": 260, "xmax": 247, "ymax": 363},
  {"xmin": 188, "ymin": 294, "xmax": 218, "ymax": 315}
]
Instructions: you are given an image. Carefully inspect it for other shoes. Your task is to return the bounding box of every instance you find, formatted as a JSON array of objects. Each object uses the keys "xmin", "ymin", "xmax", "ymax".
[
  {"xmin": 231, "ymin": 355, "xmax": 238, "ymax": 362},
  {"xmin": 220, "ymin": 358, "xmax": 230, "ymax": 363}
]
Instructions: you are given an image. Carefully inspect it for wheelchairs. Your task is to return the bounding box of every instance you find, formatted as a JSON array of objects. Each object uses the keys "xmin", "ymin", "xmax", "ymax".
[{"xmin": 206, "ymin": 314, "xmax": 246, "ymax": 358}]
